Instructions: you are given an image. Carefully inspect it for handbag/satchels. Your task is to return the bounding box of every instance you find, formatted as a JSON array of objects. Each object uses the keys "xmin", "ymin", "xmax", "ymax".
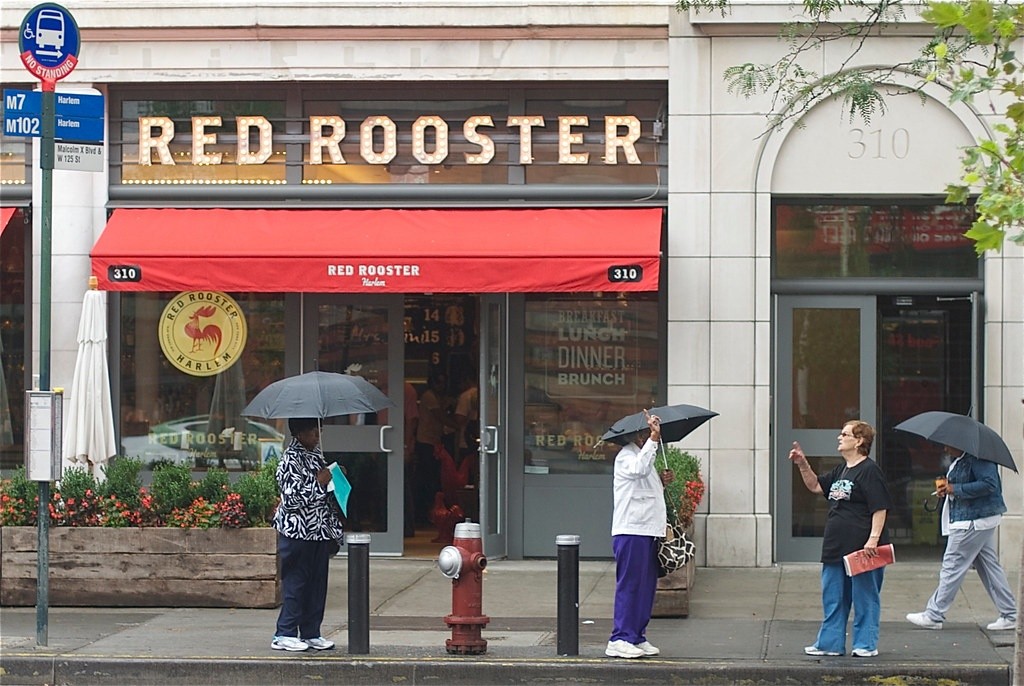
[{"xmin": 652, "ymin": 523, "xmax": 697, "ymax": 574}]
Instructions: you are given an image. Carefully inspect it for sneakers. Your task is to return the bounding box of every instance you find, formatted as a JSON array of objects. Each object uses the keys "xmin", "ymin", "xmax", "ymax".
[
  {"xmin": 850, "ymin": 647, "xmax": 879, "ymax": 657},
  {"xmin": 803, "ymin": 646, "xmax": 839, "ymax": 656},
  {"xmin": 634, "ymin": 641, "xmax": 660, "ymax": 656},
  {"xmin": 605, "ymin": 639, "xmax": 645, "ymax": 658},
  {"xmin": 905, "ymin": 611, "xmax": 943, "ymax": 630},
  {"xmin": 300, "ymin": 635, "xmax": 336, "ymax": 650},
  {"xmin": 986, "ymin": 616, "xmax": 1017, "ymax": 630},
  {"xmin": 270, "ymin": 636, "xmax": 309, "ymax": 651}
]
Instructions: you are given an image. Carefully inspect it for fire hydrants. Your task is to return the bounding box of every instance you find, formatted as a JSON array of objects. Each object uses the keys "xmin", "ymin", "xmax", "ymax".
[{"xmin": 438, "ymin": 518, "xmax": 490, "ymax": 656}]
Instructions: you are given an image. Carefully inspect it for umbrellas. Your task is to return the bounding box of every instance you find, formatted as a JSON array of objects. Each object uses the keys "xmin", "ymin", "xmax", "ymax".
[
  {"xmin": 240, "ymin": 358, "xmax": 394, "ymax": 469},
  {"xmin": 893, "ymin": 406, "xmax": 1020, "ymax": 513},
  {"xmin": 205, "ymin": 357, "xmax": 246, "ymax": 452},
  {"xmin": 66, "ymin": 275, "xmax": 116, "ymax": 476},
  {"xmin": 603, "ymin": 404, "xmax": 720, "ymax": 470}
]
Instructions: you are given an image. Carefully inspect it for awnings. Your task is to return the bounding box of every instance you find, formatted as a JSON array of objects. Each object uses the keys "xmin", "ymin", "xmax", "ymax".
[
  {"xmin": 0, "ymin": 207, "xmax": 16, "ymax": 238},
  {"xmin": 89, "ymin": 208, "xmax": 666, "ymax": 292}
]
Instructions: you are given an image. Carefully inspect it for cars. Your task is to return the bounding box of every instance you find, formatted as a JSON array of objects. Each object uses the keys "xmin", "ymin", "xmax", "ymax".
[{"xmin": 120, "ymin": 415, "xmax": 285, "ymax": 470}]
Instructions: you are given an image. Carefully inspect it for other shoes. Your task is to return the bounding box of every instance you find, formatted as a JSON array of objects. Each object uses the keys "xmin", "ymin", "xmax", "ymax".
[{"xmin": 404, "ymin": 528, "xmax": 414, "ymax": 537}]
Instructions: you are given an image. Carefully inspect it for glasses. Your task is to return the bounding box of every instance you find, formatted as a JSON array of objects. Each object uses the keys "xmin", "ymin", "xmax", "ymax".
[{"xmin": 839, "ymin": 432, "xmax": 854, "ymax": 437}]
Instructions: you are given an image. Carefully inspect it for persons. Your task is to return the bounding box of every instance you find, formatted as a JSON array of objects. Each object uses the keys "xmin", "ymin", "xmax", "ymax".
[
  {"xmin": 788, "ymin": 420, "xmax": 892, "ymax": 658},
  {"xmin": 271, "ymin": 418, "xmax": 346, "ymax": 651},
  {"xmin": 605, "ymin": 408, "xmax": 674, "ymax": 658},
  {"xmin": 374, "ymin": 363, "xmax": 480, "ymax": 539},
  {"xmin": 907, "ymin": 445, "xmax": 1018, "ymax": 630}
]
